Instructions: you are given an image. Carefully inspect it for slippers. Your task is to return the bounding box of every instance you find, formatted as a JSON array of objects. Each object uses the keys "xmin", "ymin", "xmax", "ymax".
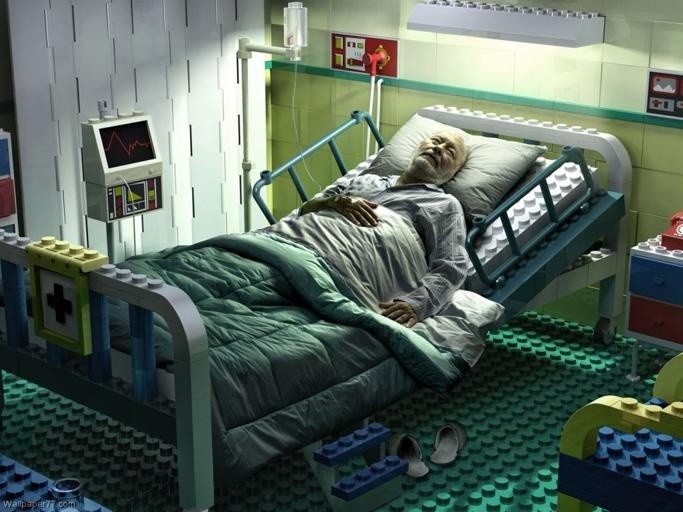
[
  {"xmin": 388, "ymin": 433, "xmax": 429, "ymax": 479},
  {"xmin": 430, "ymin": 422, "xmax": 466, "ymax": 465}
]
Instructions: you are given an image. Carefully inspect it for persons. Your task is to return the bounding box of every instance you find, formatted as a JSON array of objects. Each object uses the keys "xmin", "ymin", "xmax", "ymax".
[{"xmin": 299, "ymin": 130, "xmax": 468, "ymax": 326}]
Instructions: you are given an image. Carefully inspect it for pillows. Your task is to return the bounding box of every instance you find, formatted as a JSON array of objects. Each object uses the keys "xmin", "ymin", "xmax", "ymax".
[{"xmin": 359, "ymin": 113, "xmax": 548, "ymax": 232}]
